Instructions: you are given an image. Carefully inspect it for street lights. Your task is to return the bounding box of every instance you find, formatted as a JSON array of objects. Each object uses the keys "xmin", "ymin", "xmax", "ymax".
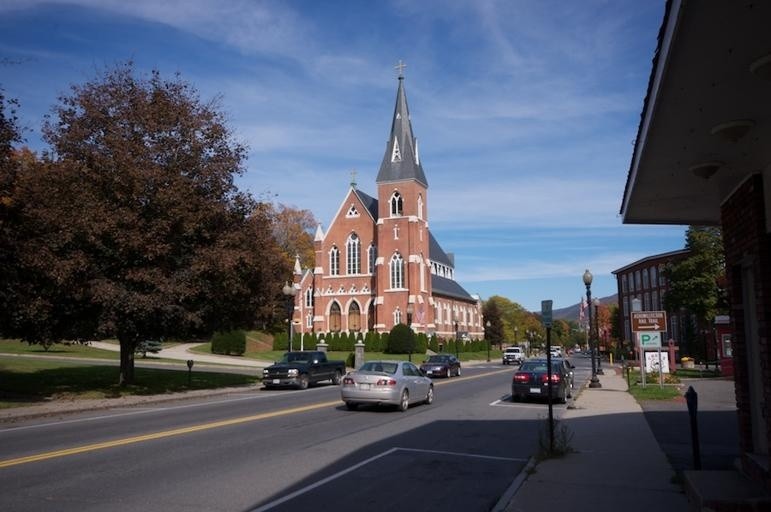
[
  {"xmin": 485, "ymin": 319, "xmax": 491, "ymax": 362},
  {"xmin": 592, "ymin": 295, "xmax": 605, "ymax": 375},
  {"xmin": 280, "ymin": 279, "xmax": 297, "ymax": 352},
  {"xmin": 454, "ymin": 315, "xmax": 460, "ymax": 358},
  {"xmin": 581, "ymin": 267, "xmax": 602, "ymax": 389},
  {"xmin": 513, "ymin": 327, "xmax": 545, "ymax": 356},
  {"xmin": 406, "ymin": 302, "xmax": 413, "ymax": 362}
]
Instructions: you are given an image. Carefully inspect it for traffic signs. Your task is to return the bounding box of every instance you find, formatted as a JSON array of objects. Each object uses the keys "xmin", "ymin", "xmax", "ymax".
[{"xmin": 630, "ymin": 310, "xmax": 666, "ymax": 333}]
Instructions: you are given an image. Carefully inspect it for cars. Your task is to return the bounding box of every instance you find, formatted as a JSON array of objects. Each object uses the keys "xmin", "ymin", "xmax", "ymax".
[
  {"xmin": 532, "ymin": 356, "xmax": 575, "ymax": 388},
  {"xmin": 511, "ymin": 359, "xmax": 572, "ymax": 404},
  {"xmin": 418, "ymin": 353, "xmax": 461, "ymax": 379},
  {"xmin": 548, "ymin": 345, "xmax": 592, "ymax": 358},
  {"xmin": 338, "ymin": 359, "xmax": 435, "ymax": 416}
]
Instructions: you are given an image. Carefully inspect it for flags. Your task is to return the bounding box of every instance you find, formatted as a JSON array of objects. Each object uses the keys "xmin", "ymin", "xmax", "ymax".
[
  {"xmin": 582, "ymin": 298, "xmax": 588, "ymax": 310},
  {"xmin": 579, "ymin": 305, "xmax": 585, "ymax": 320}
]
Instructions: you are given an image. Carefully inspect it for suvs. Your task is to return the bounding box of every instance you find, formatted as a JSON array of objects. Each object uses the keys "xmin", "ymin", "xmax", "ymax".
[{"xmin": 502, "ymin": 345, "xmax": 525, "ymax": 365}]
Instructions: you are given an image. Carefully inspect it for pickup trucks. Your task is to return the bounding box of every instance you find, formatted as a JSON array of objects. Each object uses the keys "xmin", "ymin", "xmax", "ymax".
[{"xmin": 258, "ymin": 351, "xmax": 347, "ymax": 392}]
